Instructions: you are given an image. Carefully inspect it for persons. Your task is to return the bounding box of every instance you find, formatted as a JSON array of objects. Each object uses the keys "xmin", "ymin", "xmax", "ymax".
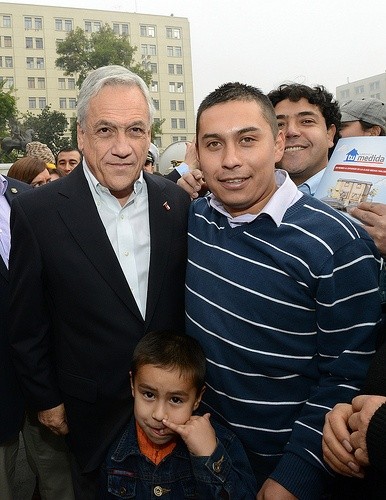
[
  {"xmin": 279, "ymin": 83, "xmax": 386, "ymax": 259},
  {"xmin": 322, "ymin": 345, "xmax": 386, "ymax": 500},
  {"xmin": 74, "ymin": 328, "xmax": 258, "ymax": 500},
  {"xmin": 0, "ymin": 146, "xmax": 81, "ymax": 500},
  {"xmin": 6, "ymin": 64, "xmax": 191, "ymax": 500},
  {"xmin": 183, "ymin": 82, "xmax": 385, "ymax": 499},
  {"xmin": 144, "ymin": 135, "xmax": 199, "ymax": 182}
]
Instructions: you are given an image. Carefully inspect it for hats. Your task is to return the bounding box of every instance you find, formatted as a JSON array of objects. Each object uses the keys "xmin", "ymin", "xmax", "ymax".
[
  {"xmin": 339, "ymin": 98, "xmax": 386, "ymax": 134},
  {"xmin": 146, "ymin": 142, "xmax": 159, "ymax": 166}
]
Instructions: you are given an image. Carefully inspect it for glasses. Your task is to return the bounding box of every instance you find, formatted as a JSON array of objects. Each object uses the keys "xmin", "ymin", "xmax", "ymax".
[{"xmin": 145, "ymin": 161, "xmax": 150, "ymax": 166}]
